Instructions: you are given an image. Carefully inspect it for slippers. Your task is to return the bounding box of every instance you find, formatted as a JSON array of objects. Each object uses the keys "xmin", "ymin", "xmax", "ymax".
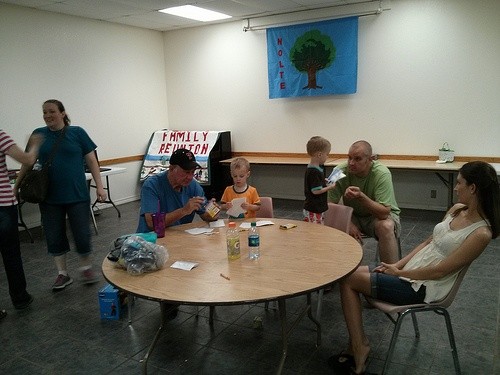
[{"xmin": 327, "ymin": 350, "xmax": 379, "ymax": 375}]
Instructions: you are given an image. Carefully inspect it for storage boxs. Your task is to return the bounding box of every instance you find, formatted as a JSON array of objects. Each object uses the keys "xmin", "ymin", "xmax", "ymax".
[{"xmin": 97, "ymin": 282, "xmax": 136, "ymax": 319}]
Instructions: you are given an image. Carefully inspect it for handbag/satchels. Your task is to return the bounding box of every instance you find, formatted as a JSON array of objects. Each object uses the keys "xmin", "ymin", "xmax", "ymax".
[{"xmin": 19, "ymin": 169, "xmax": 46, "ymax": 203}]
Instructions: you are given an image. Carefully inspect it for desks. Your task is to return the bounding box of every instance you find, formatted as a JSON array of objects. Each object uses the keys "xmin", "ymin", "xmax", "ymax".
[
  {"xmin": 216, "ymin": 152, "xmax": 339, "ymax": 183},
  {"xmin": 9, "ymin": 163, "xmax": 128, "ymax": 242},
  {"xmin": 321, "ymin": 155, "xmax": 465, "ymax": 213},
  {"xmin": 100, "ymin": 216, "xmax": 361, "ymax": 375}
]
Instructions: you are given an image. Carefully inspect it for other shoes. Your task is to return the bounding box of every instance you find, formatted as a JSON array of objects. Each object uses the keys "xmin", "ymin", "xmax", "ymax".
[
  {"xmin": 14, "ymin": 293, "xmax": 34, "ymax": 310},
  {"xmin": 0, "ymin": 309, "xmax": 7, "ymax": 319}
]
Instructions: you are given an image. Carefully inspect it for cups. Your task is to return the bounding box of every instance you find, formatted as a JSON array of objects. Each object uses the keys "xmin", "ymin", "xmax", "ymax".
[{"xmin": 150, "ymin": 211, "xmax": 166, "ymax": 238}]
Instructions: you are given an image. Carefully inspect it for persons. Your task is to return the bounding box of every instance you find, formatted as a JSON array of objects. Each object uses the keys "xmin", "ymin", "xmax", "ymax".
[
  {"xmin": 339, "ymin": 161, "xmax": 500, "ymax": 375},
  {"xmin": 301, "ymin": 136, "xmax": 336, "ymax": 226},
  {"xmin": 0, "ymin": 129, "xmax": 43, "ymax": 321},
  {"xmin": 14, "ymin": 99, "xmax": 108, "ymax": 289},
  {"xmin": 327, "ymin": 140, "xmax": 403, "ymax": 265},
  {"xmin": 221, "ymin": 157, "xmax": 262, "ymax": 221},
  {"xmin": 134, "ymin": 147, "xmax": 221, "ymax": 235}
]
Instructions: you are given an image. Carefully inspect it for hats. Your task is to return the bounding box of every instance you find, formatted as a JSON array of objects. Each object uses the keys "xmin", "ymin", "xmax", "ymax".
[{"xmin": 169, "ymin": 149, "xmax": 202, "ymax": 171}]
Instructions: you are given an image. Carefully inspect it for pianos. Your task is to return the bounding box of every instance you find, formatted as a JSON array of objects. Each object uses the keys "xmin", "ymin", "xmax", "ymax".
[{"xmin": 138, "ymin": 129, "xmax": 234, "ymax": 201}]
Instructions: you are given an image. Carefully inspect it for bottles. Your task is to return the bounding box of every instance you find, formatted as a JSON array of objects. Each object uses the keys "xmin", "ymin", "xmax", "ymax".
[
  {"xmin": 225, "ymin": 222, "xmax": 241, "ymax": 261},
  {"xmin": 248, "ymin": 222, "xmax": 260, "ymax": 260}
]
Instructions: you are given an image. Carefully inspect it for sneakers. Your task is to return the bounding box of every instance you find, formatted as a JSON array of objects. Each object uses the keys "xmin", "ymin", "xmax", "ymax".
[
  {"xmin": 51, "ymin": 274, "xmax": 73, "ymax": 289},
  {"xmin": 81, "ymin": 267, "xmax": 97, "ymax": 284}
]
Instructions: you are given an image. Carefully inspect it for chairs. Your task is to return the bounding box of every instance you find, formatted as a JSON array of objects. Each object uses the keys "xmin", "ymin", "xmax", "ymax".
[
  {"xmin": 315, "ymin": 200, "xmax": 354, "ymax": 329},
  {"xmin": 252, "ymin": 196, "xmax": 276, "ymax": 220},
  {"xmin": 365, "ymin": 258, "xmax": 473, "ymax": 375},
  {"xmin": 360, "ymin": 230, "xmax": 404, "ymax": 263}
]
